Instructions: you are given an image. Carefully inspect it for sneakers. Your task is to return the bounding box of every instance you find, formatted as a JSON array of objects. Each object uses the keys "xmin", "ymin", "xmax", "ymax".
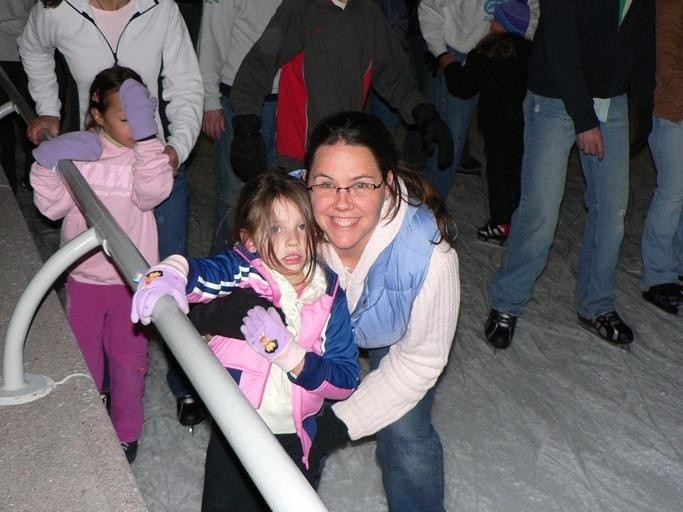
[
  {"xmin": 577, "ymin": 311, "xmax": 633, "ymax": 344},
  {"xmin": 485, "ymin": 308, "xmax": 518, "ymax": 348},
  {"xmin": 477, "ymin": 216, "xmax": 511, "ymax": 247},
  {"xmin": 643, "ymin": 283, "xmax": 682, "ymax": 316},
  {"xmin": 455, "ymin": 153, "xmax": 482, "ymax": 172}
]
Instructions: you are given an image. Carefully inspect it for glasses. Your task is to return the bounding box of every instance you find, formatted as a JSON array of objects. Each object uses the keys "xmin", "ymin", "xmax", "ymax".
[{"xmin": 304, "ymin": 167, "xmax": 389, "ymax": 198}]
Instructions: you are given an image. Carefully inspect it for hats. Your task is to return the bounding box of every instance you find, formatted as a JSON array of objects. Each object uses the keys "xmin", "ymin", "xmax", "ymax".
[{"xmin": 493, "ymin": 0, "xmax": 530, "ymax": 37}]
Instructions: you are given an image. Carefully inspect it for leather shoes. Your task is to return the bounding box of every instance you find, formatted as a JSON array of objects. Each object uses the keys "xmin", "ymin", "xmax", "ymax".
[{"xmin": 178, "ymin": 394, "xmax": 208, "ymax": 426}]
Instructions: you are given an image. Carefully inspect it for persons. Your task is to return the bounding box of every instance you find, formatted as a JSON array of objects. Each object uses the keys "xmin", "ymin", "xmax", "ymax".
[
  {"xmin": 0, "ymin": 0, "xmax": 683, "ymax": 427},
  {"xmin": 28, "ymin": 65, "xmax": 174, "ymax": 465},
  {"xmin": 130, "ymin": 110, "xmax": 460, "ymax": 511},
  {"xmin": 188, "ymin": 171, "xmax": 359, "ymax": 511}
]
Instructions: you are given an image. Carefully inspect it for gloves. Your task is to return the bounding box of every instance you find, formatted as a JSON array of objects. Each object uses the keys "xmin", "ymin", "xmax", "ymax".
[
  {"xmin": 412, "ymin": 104, "xmax": 454, "ymax": 171},
  {"xmin": 119, "ymin": 79, "xmax": 158, "ymax": 140},
  {"xmin": 238, "ymin": 305, "xmax": 308, "ymax": 374},
  {"xmin": 131, "ymin": 254, "xmax": 191, "ymax": 326},
  {"xmin": 31, "ymin": 131, "xmax": 103, "ymax": 171},
  {"xmin": 309, "ymin": 404, "xmax": 349, "ymax": 479},
  {"xmin": 187, "ymin": 287, "xmax": 287, "ymax": 341},
  {"xmin": 230, "ymin": 114, "xmax": 267, "ymax": 184}
]
